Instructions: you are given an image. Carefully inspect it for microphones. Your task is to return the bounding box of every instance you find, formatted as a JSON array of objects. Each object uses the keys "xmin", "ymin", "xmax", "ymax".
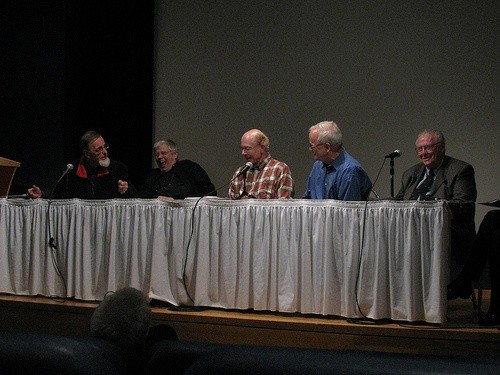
[
  {"xmin": 58, "ymin": 164, "xmax": 74, "ymax": 182},
  {"xmin": 237, "ymin": 162, "xmax": 253, "ymax": 176},
  {"xmin": 385, "ymin": 150, "xmax": 401, "ymax": 158}
]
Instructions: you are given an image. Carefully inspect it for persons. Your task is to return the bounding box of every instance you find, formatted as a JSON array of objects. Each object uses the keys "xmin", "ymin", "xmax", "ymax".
[
  {"xmin": 301, "ymin": 121, "xmax": 372, "ymax": 201},
  {"xmin": 78, "ymin": 287, "xmax": 179, "ymax": 357},
  {"xmin": 395, "ymin": 129, "xmax": 478, "ymax": 256},
  {"xmin": 447, "ymin": 209, "xmax": 500, "ymax": 327},
  {"xmin": 227, "ymin": 128, "xmax": 295, "ymax": 200},
  {"xmin": 27, "ymin": 131, "xmax": 136, "ymax": 199},
  {"xmin": 135, "ymin": 138, "xmax": 218, "ymax": 200}
]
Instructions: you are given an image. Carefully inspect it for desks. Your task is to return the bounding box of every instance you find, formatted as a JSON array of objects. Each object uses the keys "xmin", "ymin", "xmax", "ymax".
[{"xmin": 0, "ymin": 197, "xmax": 458, "ymax": 325}]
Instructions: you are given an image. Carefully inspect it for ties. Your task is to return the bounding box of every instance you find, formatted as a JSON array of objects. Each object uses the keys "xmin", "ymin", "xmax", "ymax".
[{"xmin": 412, "ymin": 170, "xmax": 435, "ymax": 200}]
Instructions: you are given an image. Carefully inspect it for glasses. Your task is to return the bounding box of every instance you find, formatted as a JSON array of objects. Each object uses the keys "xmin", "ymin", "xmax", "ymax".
[
  {"xmin": 416, "ymin": 141, "xmax": 441, "ymax": 151},
  {"xmin": 310, "ymin": 144, "xmax": 321, "ymax": 149},
  {"xmin": 242, "ymin": 144, "xmax": 260, "ymax": 150},
  {"xmin": 88, "ymin": 144, "xmax": 109, "ymax": 152}
]
